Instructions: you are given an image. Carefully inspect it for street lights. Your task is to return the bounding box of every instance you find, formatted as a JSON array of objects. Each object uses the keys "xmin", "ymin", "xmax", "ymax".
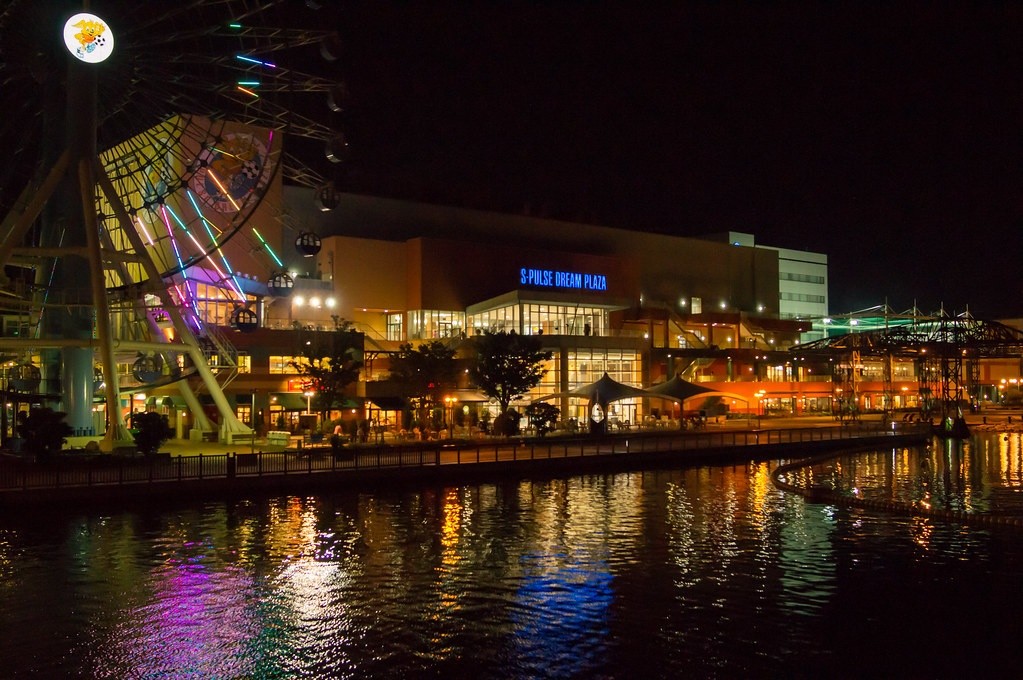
[
  {"xmin": 444, "ymin": 397, "xmax": 458, "ymax": 438},
  {"xmin": 249, "ymin": 388, "xmax": 260, "ymax": 454},
  {"xmin": 754, "ymin": 392, "xmax": 764, "ymax": 429},
  {"xmin": 902, "ymin": 387, "xmax": 908, "ymax": 407},
  {"xmin": 304, "ymin": 391, "xmax": 315, "ymax": 414}
]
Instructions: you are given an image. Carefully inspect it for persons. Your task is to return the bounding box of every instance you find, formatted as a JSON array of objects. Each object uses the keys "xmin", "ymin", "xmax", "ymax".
[
  {"xmin": 749, "ymin": 338, "xmax": 756, "ymax": 348},
  {"xmin": 333, "ymin": 423, "xmax": 343, "ymax": 436},
  {"xmin": 699, "ymin": 408, "xmax": 706, "ymax": 417},
  {"xmin": 350, "ymin": 422, "xmax": 358, "ymax": 443}
]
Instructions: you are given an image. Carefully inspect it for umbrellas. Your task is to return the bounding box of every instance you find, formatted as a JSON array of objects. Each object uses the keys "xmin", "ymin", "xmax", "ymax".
[{"xmin": 530, "ymin": 372, "xmax": 749, "ymax": 432}]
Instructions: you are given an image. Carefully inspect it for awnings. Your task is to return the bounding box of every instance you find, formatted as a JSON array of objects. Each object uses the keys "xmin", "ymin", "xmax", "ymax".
[
  {"xmin": 269, "ymin": 392, "xmax": 359, "ymax": 410},
  {"xmin": 368, "ymin": 397, "xmax": 416, "ymax": 410},
  {"xmin": 450, "ymin": 391, "xmax": 489, "ymax": 402}
]
